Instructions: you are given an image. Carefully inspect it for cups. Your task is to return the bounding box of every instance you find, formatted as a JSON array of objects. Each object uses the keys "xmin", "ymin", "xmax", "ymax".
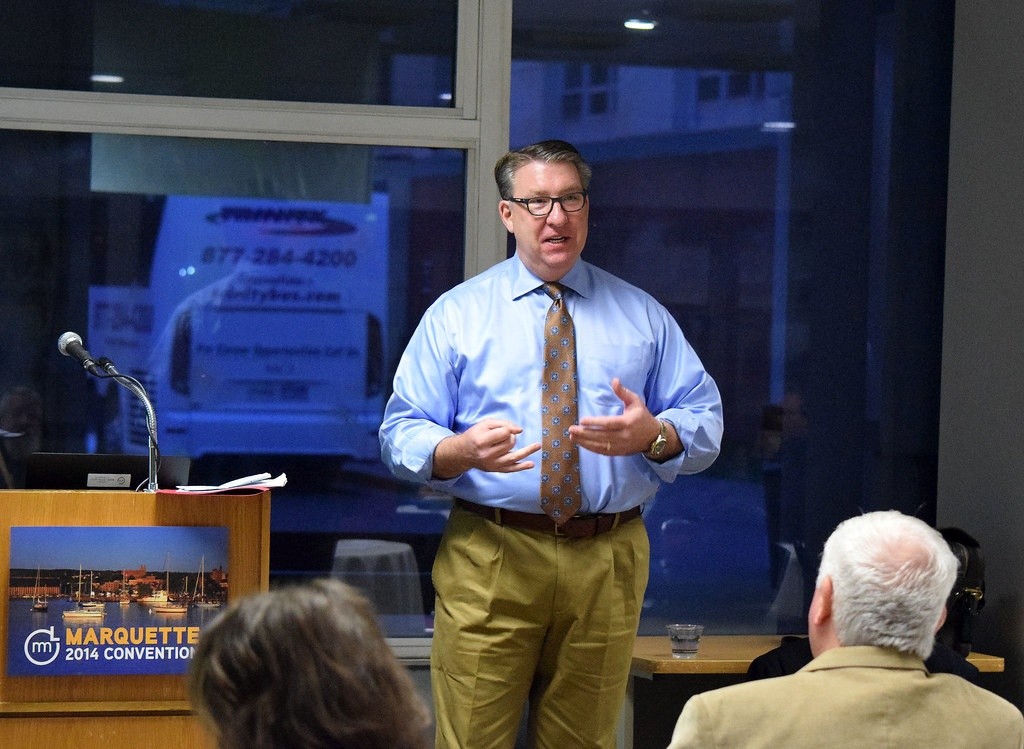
[{"xmin": 667, "ymin": 621, "xmax": 703, "ymax": 658}]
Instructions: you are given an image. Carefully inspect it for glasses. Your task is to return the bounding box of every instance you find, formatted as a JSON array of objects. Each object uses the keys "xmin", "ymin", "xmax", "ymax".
[{"xmin": 509, "ymin": 189, "xmax": 591, "ymax": 216}]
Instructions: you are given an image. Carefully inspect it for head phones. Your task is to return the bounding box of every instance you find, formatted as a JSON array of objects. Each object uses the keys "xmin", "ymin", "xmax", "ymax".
[{"xmin": 934, "ymin": 526, "xmax": 987, "ymax": 629}]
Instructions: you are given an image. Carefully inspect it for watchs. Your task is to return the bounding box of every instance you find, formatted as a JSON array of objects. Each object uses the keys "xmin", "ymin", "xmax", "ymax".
[{"xmin": 641, "ymin": 421, "xmax": 669, "ymax": 462}]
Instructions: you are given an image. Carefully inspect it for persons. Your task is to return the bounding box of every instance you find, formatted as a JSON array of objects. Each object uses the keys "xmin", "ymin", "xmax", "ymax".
[
  {"xmin": 663, "ymin": 511, "xmax": 1022, "ymax": 749},
  {"xmin": 374, "ymin": 136, "xmax": 725, "ymax": 749},
  {"xmin": 188, "ymin": 581, "xmax": 438, "ymax": 749}
]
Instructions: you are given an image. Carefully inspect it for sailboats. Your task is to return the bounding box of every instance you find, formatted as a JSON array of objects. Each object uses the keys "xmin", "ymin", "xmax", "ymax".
[{"xmin": 30, "ymin": 551, "xmax": 221, "ymax": 621}]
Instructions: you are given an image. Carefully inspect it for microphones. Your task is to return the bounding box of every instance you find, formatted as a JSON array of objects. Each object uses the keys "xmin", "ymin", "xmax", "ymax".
[{"xmin": 58, "ymin": 331, "xmax": 98, "ymax": 376}]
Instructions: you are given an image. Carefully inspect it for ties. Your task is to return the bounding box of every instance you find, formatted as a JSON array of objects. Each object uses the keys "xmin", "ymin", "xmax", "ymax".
[{"xmin": 541, "ymin": 282, "xmax": 583, "ymax": 526}]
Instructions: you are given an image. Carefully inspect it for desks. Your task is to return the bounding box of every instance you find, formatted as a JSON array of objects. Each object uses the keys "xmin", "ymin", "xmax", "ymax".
[{"xmin": 618, "ymin": 634, "xmax": 1005, "ymax": 749}]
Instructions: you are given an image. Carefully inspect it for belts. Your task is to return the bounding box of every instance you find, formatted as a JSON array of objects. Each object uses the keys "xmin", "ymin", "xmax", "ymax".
[{"xmin": 455, "ymin": 496, "xmax": 641, "ymax": 540}]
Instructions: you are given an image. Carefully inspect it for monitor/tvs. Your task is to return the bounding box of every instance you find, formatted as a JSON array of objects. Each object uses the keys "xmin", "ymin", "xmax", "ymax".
[{"xmin": 27, "ymin": 452, "xmax": 191, "ymax": 490}]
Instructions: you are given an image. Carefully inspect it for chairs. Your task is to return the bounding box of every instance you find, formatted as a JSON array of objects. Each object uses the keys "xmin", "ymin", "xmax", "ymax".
[{"xmin": 642, "ymin": 470, "xmax": 784, "ymax": 633}]
